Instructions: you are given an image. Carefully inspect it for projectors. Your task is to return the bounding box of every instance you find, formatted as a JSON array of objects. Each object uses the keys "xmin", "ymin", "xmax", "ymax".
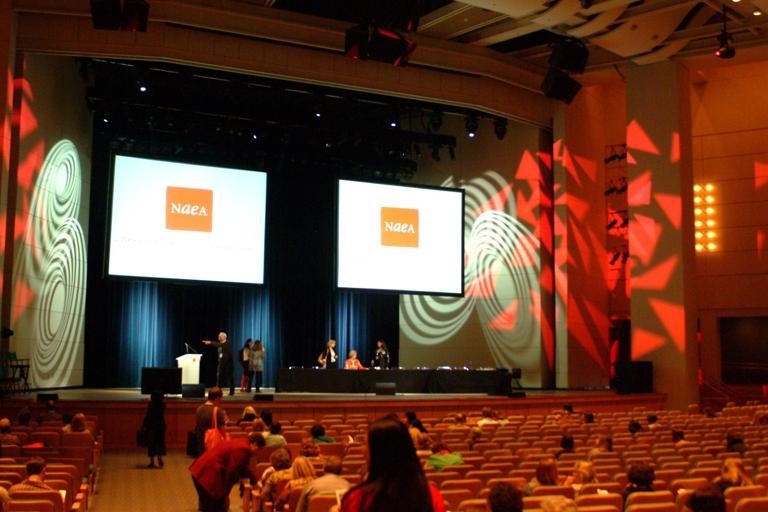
[{"xmin": 345, "ymin": 26, "xmax": 416, "ymax": 67}]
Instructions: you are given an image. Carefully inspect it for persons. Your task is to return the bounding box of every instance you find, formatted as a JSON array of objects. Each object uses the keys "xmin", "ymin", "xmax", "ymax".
[
  {"xmin": 321, "ymin": 338, "xmax": 339, "ymax": 369},
  {"xmin": 226, "ymin": 403, "xmax": 767, "ymax": 511},
  {"xmin": 343, "ymin": 349, "xmax": 369, "ymax": 370},
  {"xmin": 238, "ymin": 337, "xmax": 252, "ymax": 392},
  {"xmin": 369, "ymin": 338, "xmax": 390, "ymax": 369},
  {"xmin": 202, "ymin": 331, "xmax": 235, "ymax": 397},
  {"xmin": 0, "ymin": 395, "xmax": 98, "ymax": 512},
  {"xmin": 143, "ymin": 390, "xmax": 167, "ymax": 467},
  {"xmin": 196, "ymin": 387, "xmax": 226, "ymax": 455},
  {"xmin": 188, "ymin": 433, "xmax": 269, "ymax": 512},
  {"xmin": 244, "ymin": 339, "xmax": 264, "ymax": 393}
]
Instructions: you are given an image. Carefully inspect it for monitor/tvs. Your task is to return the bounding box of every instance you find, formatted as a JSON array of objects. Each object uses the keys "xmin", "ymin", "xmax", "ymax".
[{"xmin": 141, "ymin": 366, "xmax": 181, "ymax": 398}]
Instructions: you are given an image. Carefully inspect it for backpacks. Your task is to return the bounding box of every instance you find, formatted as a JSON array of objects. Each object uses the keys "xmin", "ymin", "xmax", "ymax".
[{"xmin": 203, "ymin": 405, "xmax": 230, "ymax": 454}]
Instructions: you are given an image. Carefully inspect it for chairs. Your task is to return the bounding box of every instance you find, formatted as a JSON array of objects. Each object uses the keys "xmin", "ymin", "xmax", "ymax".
[
  {"xmin": 2, "ymin": 358, "xmax": 32, "ymax": 395},
  {"xmin": 0, "ymin": 409, "xmax": 104, "ymax": 510}
]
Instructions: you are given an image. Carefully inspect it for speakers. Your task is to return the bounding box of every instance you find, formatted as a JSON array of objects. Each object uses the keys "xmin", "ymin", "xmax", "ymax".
[
  {"xmin": 90, "ymin": 0, "xmax": 149, "ymax": 32},
  {"xmin": 253, "ymin": 394, "xmax": 274, "ymax": 401},
  {"xmin": 183, "ymin": 385, "xmax": 205, "ymax": 398},
  {"xmin": 376, "ymin": 381, "xmax": 397, "ymax": 394},
  {"xmin": 36, "ymin": 394, "xmax": 58, "ymax": 400},
  {"xmin": 552, "ymin": 38, "xmax": 589, "ymax": 71},
  {"xmin": 543, "ymin": 70, "xmax": 581, "ymax": 104}
]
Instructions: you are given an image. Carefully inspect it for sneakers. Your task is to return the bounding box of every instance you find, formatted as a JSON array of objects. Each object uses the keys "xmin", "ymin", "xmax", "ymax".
[
  {"xmin": 158, "ymin": 460, "xmax": 164, "ymax": 467},
  {"xmin": 147, "ymin": 463, "xmax": 153, "ymax": 468}
]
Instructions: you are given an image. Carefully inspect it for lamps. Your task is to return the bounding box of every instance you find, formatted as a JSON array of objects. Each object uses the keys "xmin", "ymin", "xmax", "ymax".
[
  {"xmin": 602, "ymin": 144, "xmax": 627, "ymax": 266},
  {"xmin": 714, "ymin": 6, "xmax": 735, "ymax": 61}
]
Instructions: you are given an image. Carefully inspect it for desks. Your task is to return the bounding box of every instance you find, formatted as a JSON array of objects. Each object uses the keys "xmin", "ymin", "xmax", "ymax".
[{"xmin": 273, "ymin": 368, "xmax": 522, "ymax": 395}]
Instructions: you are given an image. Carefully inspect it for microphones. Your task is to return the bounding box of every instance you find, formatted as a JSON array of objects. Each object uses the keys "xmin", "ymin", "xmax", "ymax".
[{"xmin": 184, "ymin": 341, "xmax": 189, "ymax": 347}]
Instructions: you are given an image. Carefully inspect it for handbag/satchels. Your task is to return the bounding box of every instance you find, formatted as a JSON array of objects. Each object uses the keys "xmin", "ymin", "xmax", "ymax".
[
  {"xmin": 135, "ymin": 425, "xmax": 154, "ymax": 448},
  {"xmin": 316, "ymin": 352, "xmax": 326, "ymax": 365}
]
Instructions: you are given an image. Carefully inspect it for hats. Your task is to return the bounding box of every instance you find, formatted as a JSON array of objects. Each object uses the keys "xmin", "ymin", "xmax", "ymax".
[{"xmin": 0, "ymin": 417, "xmax": 11, "ymax": 429}]
